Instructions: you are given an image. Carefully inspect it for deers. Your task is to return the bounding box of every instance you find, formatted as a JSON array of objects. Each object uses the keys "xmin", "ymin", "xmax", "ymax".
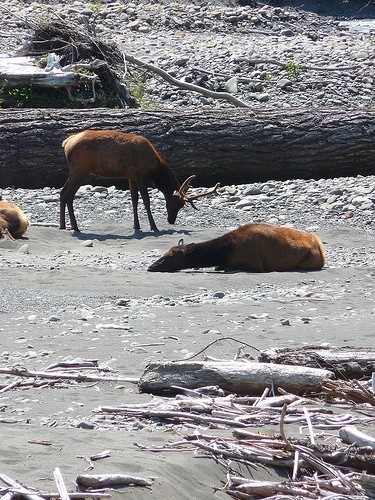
[
  {"xmin": 57, "ymin": 128, "xmax": 221, "ymax": 234},
  {"xmin": 148, "ymin": 223, "xmax": 326, "ymax": 274},
  {"xmin": 0, "ymin": 201, "xmax": 30, "ymax": 241}
]
